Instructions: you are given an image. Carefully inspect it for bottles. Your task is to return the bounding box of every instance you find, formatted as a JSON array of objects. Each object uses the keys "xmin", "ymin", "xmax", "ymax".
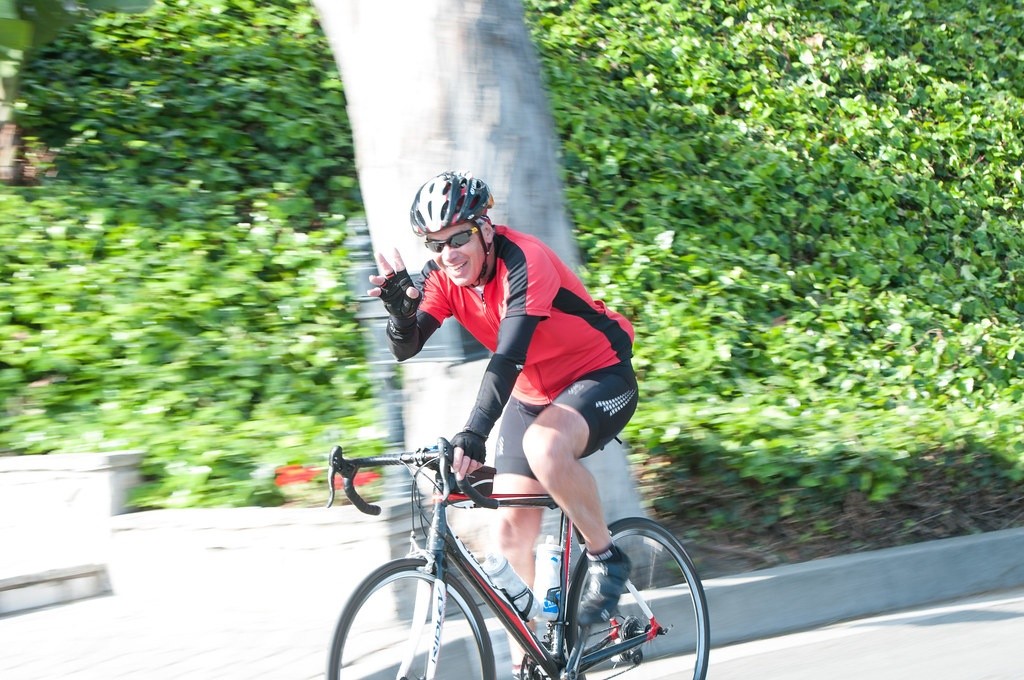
[
  {"xmin": 533, "ymin": 535, "xmax": 563, "ymax": 622},
  {"xmin": 481, "ymin": 553, "xmax": 540, "ymax": 621}
]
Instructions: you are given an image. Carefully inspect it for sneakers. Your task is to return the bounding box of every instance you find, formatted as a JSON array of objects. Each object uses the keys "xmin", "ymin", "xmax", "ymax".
[{"xmin": 576, "ymin": 546, "xmax": 632, "ymax": 627}]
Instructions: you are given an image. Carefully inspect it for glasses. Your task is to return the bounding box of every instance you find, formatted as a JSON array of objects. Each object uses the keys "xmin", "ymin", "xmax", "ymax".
[{"xmin": 424, "ymin": 227, "xmax": 481, "ymax": 254}]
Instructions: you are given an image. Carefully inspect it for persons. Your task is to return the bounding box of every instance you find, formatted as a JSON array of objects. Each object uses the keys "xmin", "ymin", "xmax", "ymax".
[{"xmin": 367, "ymin": 173, "xmax": 638, "ymax": 680}]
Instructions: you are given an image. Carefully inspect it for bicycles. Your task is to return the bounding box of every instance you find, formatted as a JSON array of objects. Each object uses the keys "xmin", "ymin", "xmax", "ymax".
[{"xmin": 327, "ymin": 437, "xmax": 711, "ymax": 680}]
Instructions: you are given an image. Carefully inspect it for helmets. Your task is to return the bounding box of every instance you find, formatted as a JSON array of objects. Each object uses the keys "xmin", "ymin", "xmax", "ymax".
[{"xmin": 410, "ymin": 170, "xmax": 495, "ymax": 238}]
